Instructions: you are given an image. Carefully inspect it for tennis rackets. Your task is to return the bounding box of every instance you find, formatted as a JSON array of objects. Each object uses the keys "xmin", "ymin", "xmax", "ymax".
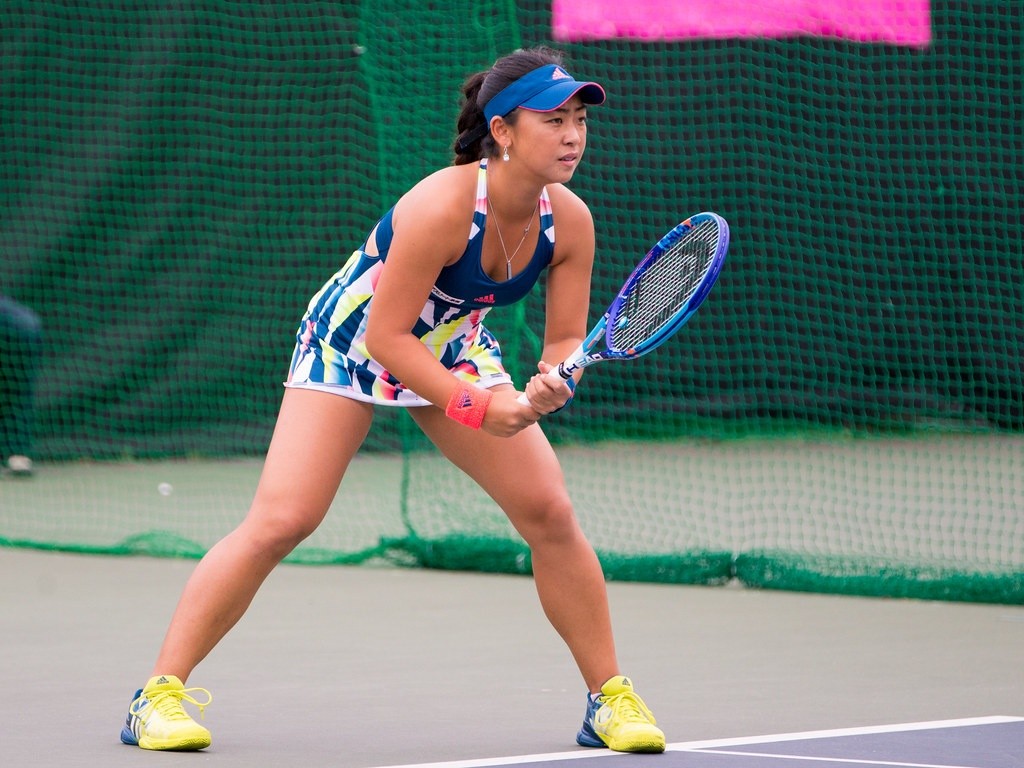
[{"xmin": 515, "ymin": 210, "xmax": 729, "ymax": 410}]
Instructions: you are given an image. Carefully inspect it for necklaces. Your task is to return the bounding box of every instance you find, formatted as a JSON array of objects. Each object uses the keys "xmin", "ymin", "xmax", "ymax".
[{"xmin": 487, "ymin": 196, "xmax": 539, "ymax": 280}]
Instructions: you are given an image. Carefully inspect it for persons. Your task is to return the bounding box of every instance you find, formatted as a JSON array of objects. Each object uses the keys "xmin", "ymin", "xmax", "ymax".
[{"xmin": 121, "ymin": 53, "xmax": 666, "ymax": 755}]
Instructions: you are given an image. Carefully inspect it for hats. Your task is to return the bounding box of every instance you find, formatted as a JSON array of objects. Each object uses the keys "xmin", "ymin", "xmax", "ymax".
[{"xmin": 482, "ymin": 64, "xmax": 606, "ymax": 130}]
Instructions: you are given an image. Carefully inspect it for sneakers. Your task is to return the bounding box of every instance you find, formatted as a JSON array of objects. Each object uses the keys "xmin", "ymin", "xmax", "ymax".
[
  {"xmin": 119, "ymin": 675, "xmax": 212, "ymax": 751},
  {"xmin": 577, "ymin": 676, "xmax": 666, "ymax": 752}
]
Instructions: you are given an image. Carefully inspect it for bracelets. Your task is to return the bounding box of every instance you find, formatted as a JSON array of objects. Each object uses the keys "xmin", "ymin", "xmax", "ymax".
[
  {"xmin": 548, "ymin": 376, "xmax": 575, "ymax": 415},
  {"xmin": 445, "ymin": 380, "xmax": 492, "ymax": 430}
]
